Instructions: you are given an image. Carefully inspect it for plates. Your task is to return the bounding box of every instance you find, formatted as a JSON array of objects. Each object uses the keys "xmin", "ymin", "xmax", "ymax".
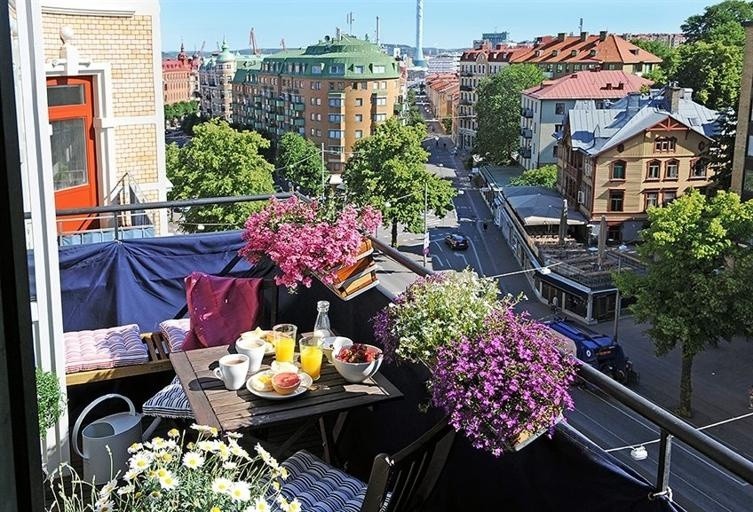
[
  {"xmin": 245, "ymin": 369, "xmax": 314, "ymax": 400},
  {"xmin": 235, "ymin": 330, "xmax": 291, "ymax": 357}
]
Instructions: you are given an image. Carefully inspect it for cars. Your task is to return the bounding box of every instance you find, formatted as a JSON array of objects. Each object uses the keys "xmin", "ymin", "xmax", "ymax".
[{"xmin": 446, "ymin": 234, "xmax": 468, "ymax": 251}]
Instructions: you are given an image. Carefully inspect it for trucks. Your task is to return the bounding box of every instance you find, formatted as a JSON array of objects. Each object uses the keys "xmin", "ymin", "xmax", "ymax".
[{"xmin": 545, "ymin": 317, "xmax": 633, "ymax": 386}]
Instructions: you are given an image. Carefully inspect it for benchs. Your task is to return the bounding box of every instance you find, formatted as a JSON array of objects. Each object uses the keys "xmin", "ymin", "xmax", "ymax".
[{"xmin": 62, "ymin": 317, "xmax": 196, "ymax": 386}]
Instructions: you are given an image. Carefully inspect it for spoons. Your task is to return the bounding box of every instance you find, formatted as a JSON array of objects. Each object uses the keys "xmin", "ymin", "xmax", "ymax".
[{"xmin": 299, "ymin": 384, "xmax": 320, "ymax": 392}]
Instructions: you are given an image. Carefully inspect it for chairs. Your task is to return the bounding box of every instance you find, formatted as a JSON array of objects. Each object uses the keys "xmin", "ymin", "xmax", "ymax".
[
  {"xmin": 139, "ymin": 271, "xmax": 280, "ymax": 445},
  {"xmin": 256, "ymin": 412, "xmax": 455, "ymax": 512}
]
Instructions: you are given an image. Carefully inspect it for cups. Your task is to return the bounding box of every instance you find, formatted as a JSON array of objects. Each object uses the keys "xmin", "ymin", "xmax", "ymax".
[
  {"xmin": 214, "ymin": 353, "xmax": 250, "ymax": 391},
  {"xmin": 273, "ymin": 323, "xmax": 298, "ymax": 365},
  {"xmin": 234, "ymin": 338, "xmax": 267, "ymax": 372},
  {"xmin": 298, "ymin": 335, "xmax": 325, "ymax": 381}
]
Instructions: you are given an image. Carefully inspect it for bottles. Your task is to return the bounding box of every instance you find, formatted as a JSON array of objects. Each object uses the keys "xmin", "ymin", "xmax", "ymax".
[{"xmin": 314, "ymin": 301, "xmax": 331, "ymax": 355}]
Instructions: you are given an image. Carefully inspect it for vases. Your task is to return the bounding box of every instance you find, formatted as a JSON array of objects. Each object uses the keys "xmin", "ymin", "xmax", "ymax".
[
  {"xmin": 326, "ymin": 238, "xmax": 374, "ymax": 296},
  {"xmin": 414, "ymin": 323, "xmax": 576, "ymax": 452}
]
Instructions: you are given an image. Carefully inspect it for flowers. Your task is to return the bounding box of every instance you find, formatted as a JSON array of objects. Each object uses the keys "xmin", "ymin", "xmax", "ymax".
[
  {"xmin": 43, "ymin": 422, "xmax": 299, "ymax": 511},
  {"xmin": 239, "ymin": 196, "xmax": 389, "ymax": 295},
  {"xmin": 368, "ymin": 264, "xmax": 582, "ymax": 455}
]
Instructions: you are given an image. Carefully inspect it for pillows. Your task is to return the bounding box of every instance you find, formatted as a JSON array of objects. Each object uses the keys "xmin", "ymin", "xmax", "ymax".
[{"xmin": 182, "ymin": 274, "xmax": 263, "ymax": 351}]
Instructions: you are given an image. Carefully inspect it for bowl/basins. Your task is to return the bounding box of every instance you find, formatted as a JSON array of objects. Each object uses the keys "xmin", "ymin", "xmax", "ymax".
[
  {"xmin": 272, "ymin": 372, "xmax": 301, "ymax": 395},
  {"xmin": 331, "ymin": 343, "xmax": 385, "ymax": 384},
  {"xmin": 320, "ymin": 335, "xmax": 354, "ymax": 364}
]
